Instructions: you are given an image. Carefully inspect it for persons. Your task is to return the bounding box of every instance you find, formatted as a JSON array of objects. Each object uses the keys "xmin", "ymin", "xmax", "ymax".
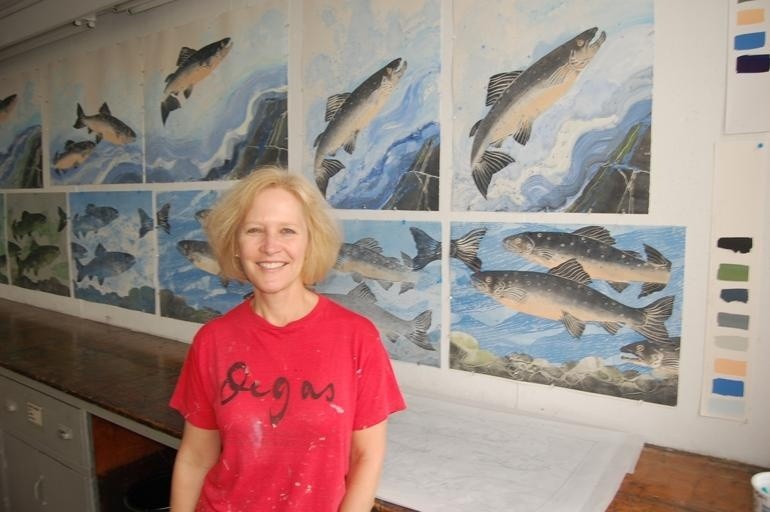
[{"xmin": 165, "ymin": 165, "xmax": 407, "ymax": 511}]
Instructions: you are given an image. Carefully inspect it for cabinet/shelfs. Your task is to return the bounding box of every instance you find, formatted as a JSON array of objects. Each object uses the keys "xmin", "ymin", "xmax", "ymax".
[{"xmin": 0, "ymin": 377, "xmax": 162, "ymax": 512}]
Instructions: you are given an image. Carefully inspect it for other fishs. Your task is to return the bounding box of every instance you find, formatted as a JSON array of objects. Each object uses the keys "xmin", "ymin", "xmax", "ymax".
[{"xmin": 0, "ymin": 23, "xmax": 679, "ymax": 372}]
